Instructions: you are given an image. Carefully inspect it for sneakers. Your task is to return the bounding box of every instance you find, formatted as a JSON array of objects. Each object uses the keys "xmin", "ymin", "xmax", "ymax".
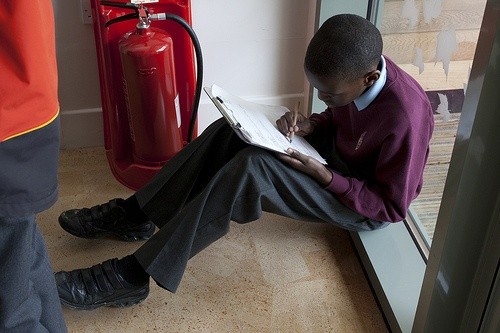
[
  {"xmin": 56, "ymin": 258, "xmax": 150, "ymax": 311},
  {"xmin": 58, "ymin": 198, "xmax": 155, "ymax": 242}
]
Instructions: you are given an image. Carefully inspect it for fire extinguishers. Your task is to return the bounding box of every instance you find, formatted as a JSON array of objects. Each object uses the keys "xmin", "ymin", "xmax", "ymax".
[{"xmin": 99, "ymin": 0, "xmax": 204, "ymax": 166}]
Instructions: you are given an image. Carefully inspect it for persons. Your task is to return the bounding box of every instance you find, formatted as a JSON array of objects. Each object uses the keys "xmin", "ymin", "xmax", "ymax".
[
  {"xmin": 54, "ymin": 14, "xmax": 434, "ymax": 310},
  {"xmin": 0, "ymin": 0, "xmax": 67, "ymax": 333}
]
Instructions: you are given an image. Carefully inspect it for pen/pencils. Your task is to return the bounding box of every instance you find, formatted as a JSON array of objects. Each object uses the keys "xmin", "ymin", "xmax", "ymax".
[{"xmin": 289, "ymin": 99, "xmax": 301, "ymax": 143}]
